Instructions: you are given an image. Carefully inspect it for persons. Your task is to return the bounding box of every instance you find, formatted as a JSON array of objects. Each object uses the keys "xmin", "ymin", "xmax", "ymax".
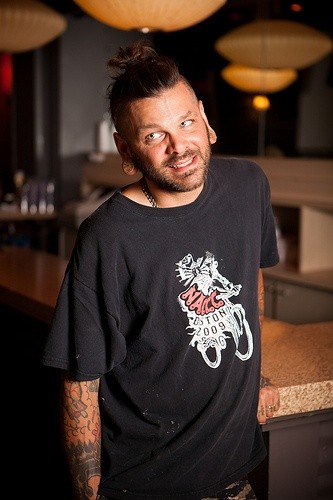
[{"xmin": 42, "ymin": 42, "xmax": 280, "ymax": 500}]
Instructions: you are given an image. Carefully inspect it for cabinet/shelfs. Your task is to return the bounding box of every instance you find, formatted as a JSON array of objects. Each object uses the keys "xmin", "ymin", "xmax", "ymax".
[{"xmin": 56, "ymin": 153, "xmax": 333, "ymax": 325}]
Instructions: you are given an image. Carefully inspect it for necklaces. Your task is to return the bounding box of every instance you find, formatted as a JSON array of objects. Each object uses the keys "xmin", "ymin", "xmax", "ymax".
[{"xmin": 141, "ymin": 177, "xmax": 160, "ymax": 209}]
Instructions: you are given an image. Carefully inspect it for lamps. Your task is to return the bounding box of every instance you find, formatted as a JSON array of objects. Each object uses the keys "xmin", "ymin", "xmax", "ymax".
[
  {"xmin": 221, "ymin": 63, "xmax": 298, "ymax": 93},
  {"xmin": 74, "ymin": 0, "xmax": 227, "ymax": 34},
  {"xmin": 0, "ymin": 0, "xmax": 68, "ymax": 53},
  {"xmin": 215, "ymin": 20, "xmax": 333, "ymax": 69}
]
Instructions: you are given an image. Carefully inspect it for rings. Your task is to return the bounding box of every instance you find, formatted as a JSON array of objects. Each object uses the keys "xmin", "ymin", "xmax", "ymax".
[{"xmin": 268, "ymin": 404, "xmax": 275, "ymax": 409}]
